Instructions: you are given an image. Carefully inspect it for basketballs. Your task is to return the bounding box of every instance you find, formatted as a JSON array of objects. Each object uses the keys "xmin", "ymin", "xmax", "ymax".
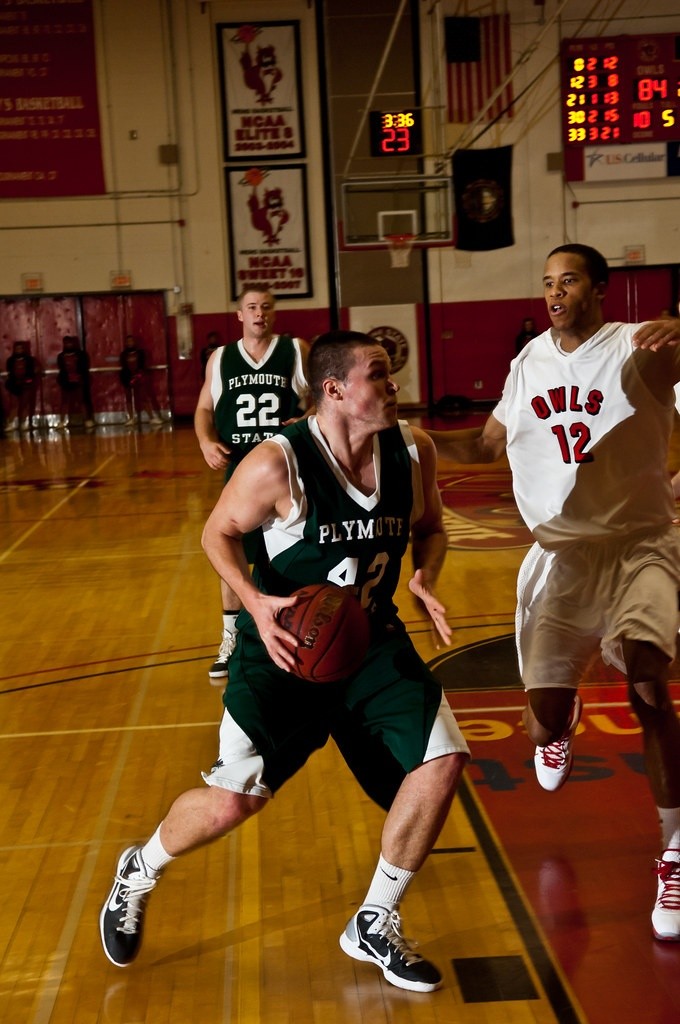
[{"xmin": 276, "ymin": 583, "xmax": 370, "ymax": 682}]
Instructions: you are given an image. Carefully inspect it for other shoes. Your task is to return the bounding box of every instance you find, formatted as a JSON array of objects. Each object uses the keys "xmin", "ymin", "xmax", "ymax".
[
  {"xmin": 56, "ymin": 423, "xmax": 63, "ymax": 430},
  {"xmin": 150, "ymin": 418, "xmax": 164, "ymax": 424},
  {"xmin": 124, "ymin": 419, "xmax": 134, "ymax": 426},
  {"xmin": 86, "ymin": 420, "xmax": 94, "ymax": 427}
]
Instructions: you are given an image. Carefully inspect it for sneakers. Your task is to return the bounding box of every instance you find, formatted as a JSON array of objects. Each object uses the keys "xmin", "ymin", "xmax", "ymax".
[
  {"xmin": 339, "ymin": 905, "xmax": 443, "ymax": 992},
  {"xmin": 534, "ymin": 695, "xmax": 582, "ymax": 791},
  {"xmin": 100, "ymin": 845, "xmax": 164, "ymax": 967},
  {"xmin": 651, "ymin": 849, "xmax": 680, "ymax": 940},
  {"xmin": 208, "ymin": 628, "xmax": 239, "ymax": 677}
]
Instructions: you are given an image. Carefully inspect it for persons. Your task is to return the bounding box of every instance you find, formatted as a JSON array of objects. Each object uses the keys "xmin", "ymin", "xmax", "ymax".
[
  {"xmin": 194, "ymin": 284, "xmax": 309, "ymax": 678},
  {"xmin": 100, "ymin": 332, "xmax": 470, "ymax": 993},
  {"xmin": 426, "ymin": 243, "xmax": 679, "ymax": 940}
]
